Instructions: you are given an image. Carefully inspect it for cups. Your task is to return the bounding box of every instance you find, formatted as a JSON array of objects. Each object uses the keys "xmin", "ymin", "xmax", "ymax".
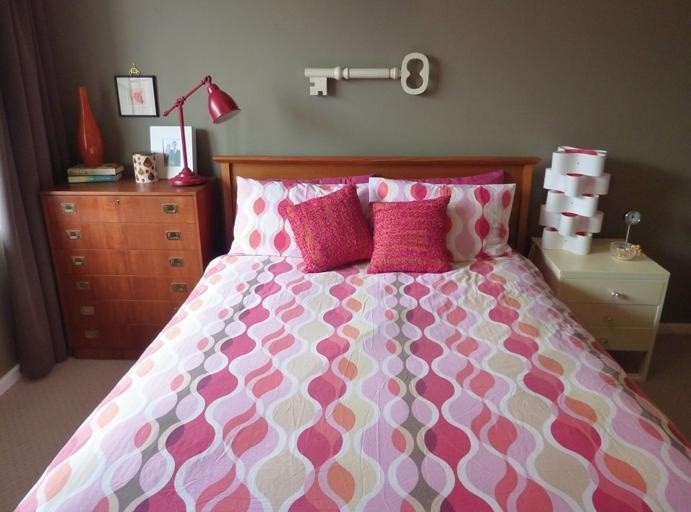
[{"xmin": 130, "ymin": 152, "xmax": 157, "ymax": 183}]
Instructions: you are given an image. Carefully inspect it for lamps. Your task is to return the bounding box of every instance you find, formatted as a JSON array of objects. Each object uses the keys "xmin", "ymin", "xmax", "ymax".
[{"xmin": 160, "ymin": 73, "xmax": 239, "ymax": 189}]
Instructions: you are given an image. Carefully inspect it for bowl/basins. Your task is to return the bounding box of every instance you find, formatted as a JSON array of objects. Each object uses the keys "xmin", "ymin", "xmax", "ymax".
[{"xmin": 609, "ymin": 241, "xmax": 640, "ymax": 261}]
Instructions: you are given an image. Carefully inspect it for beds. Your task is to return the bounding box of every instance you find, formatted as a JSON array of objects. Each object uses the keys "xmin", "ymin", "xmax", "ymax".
[{"xmin": 15, "ymin": 155, "xmax": 689, "ymax": 511}]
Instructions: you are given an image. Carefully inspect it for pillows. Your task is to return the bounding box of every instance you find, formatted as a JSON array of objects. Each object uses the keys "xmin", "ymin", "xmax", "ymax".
[{"xmin": 224, "ymin": 165, "xmax": 515, "ymax": 271}]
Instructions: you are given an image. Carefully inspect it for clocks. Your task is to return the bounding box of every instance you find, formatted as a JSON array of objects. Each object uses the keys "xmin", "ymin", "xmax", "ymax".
[{"xmin": 620, "ymin": 206, "xmax": 642, "ymax": 250}]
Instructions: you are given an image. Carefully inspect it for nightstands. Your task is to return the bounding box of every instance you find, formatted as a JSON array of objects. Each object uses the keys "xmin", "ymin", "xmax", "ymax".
[
  {"xmin": 530, "ymin": 233, "xmax": 671, "ymax": 382},
  {"xmin": 40, "ymin": 175, "xmax": 219, "ymax": 360}
]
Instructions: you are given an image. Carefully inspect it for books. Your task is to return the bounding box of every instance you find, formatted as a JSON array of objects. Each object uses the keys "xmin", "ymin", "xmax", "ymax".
[{"xmin": 66, "ymin": 163, "xmax": 124, "ymax": 182}]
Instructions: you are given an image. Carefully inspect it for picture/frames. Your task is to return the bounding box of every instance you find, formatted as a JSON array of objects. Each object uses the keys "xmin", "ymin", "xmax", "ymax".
[
  {"xmin": 114, "ymin": 75, "xmax": 159, "ymax": 118},
  {"xmin": 147, "ymin": 125, "xmax": 199, "ymax": 178}
]
